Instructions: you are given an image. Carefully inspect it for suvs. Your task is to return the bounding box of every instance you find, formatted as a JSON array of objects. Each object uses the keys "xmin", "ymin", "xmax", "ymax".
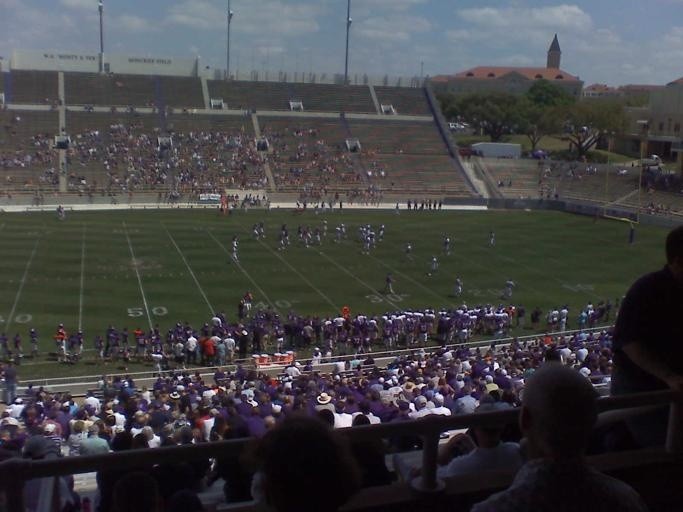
[{"xmin": 252, "ymin": 351, "xmax": 294, "ymax": 366}]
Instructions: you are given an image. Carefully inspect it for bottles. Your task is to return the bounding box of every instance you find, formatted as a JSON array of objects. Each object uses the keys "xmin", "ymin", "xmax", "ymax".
[
  {"xmin": 415, "ymin": 395, "xmax": 427, "ymax": 407},
  {"xmin": 434, "ymin": 392, "xmax": 444, "ymax": 404},
  {"xmin": 289, "ymin": 375, "xmax": 292, "ymax": 380},
  {"xmin": 169, "ymin": 391, "xmax": 180, "ymax": 399},
  {"xmin": 317, "ymin": 392, "xmax": 332, "ymax": 404},
  {"xmin": 501, "ymin": 369, "xmax": 507, "ymax": 376},
  {"xmin": 486, "ymin": 375, "xmax": 493, "ymax": 383}
]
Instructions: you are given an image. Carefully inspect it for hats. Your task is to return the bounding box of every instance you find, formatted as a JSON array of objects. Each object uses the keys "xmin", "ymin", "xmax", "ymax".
[
  {"xmin": 533, "ymin": 150, "xmax": 550, "ymax": 160},
  {"xmin": 567, "ymin": 123, "xmax": 592, "ymax": 136},
  {"xmin": 449, "ymin": 118, "xmax": 492, "ymax": 133}
]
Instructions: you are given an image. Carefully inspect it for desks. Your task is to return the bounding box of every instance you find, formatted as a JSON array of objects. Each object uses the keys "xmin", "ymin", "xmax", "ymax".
[
  {"xmin": 97, "ymin": 1, "xmax": 106, "ymax": 57},
  {"xmin": 343, "ymin": 13, "xmax": 353, "ymax": 77},
  {"xmin": 226, "ymin": 9, "xmax": 235, "ymax": 74}
]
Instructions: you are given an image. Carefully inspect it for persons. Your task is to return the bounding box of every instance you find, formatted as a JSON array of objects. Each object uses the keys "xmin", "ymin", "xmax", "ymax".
[
  {"xmin": 609, "ymin": 223, "xmax": 683, "ymax": 448},
  {"xmin": 487, "ymin": 229, "xmax": 495, "ymax": 248},
  {"xmin": 384, "ymin": 270, "xmax": 395, "ymax": 293},
  {"xmin": 442, "ymin": 236, "xmax": 451, "ymax": 256},
  {"xmin": 451, "ymin": 271, "xmax": 463, "ymax": 297},
  {"xmin": 0, "ymin": 94, "xmax": 444, "ymax": 222},
  {"xmin": 500, "ymin": 278, "xmax": 516, "ymax": 300},
  {"xmin": 495, "ymin": 150, "xmax": 676, "ymax": 216},
  {"xmin": 431, "ymin": 255, "xmax": 440, "ymax": 271},
  {"xmin": 246, "ymin": 220, "xmax": 387, "ymax": 257},
  {"xmin": 0, "ymin": 293, "xmax": 683, "ymax": 509},
  {"xmin": 406, "ymin": 240, "xmax": 413, "ymax": 254},
  {"xmin": 229, "ymin": 234, "xmax": 239, "ymax": 262}
]
